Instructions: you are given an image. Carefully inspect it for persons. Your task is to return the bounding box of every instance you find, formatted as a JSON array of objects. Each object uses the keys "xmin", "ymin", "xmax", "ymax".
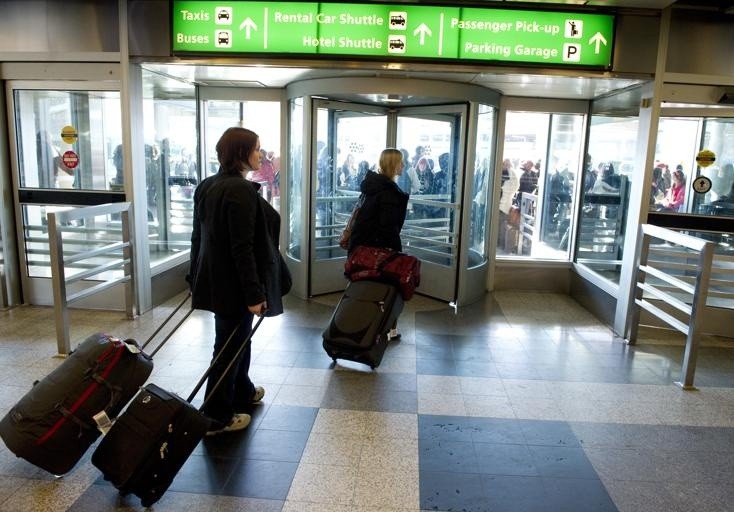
[
  {"xmin": 345, "ymin": 146, "xmax": 411, "ymax": 344},
  {"xmin": 316, "ymin": 140, "xmax": 486, "ymax": 246},
  {"xmin": 183, "ymin": 127, "xmax": 294, "ymax": 436},
  {"xmin": 110, "ymin": 139, "xmax": 281, "ymax": 223},
  {"xmin": 498, "ymin": 153, "xmax": 734, "ymax": 247}
]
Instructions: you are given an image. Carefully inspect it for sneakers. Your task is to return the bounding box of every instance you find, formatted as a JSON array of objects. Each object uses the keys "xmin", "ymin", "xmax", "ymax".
[
  {"xmin": 206, "ymin": 413, "xmax": 252, "ymax": 436},
  {"xmin": 389, "ymin": 333, "xmax": 401, "ymax": 342},
  {"xmin": 242, "ymin": 386, "xmax": 265, "ymax": 403}
]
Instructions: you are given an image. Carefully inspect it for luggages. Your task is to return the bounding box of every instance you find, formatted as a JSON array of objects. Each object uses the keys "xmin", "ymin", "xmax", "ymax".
[
  {"xmin": 91, "ymin": 306, "xmax": 272, "ymax": 508},
  {"xmin": 0, "ymin": 291, "xmax": 195, "ymax": 481},
  {"xmin": 322, "ymin": 279, "xmax": 405, "ymax": 370}
]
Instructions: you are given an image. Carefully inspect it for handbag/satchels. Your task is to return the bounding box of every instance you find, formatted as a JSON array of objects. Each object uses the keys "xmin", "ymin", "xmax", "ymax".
[
  {"xmin": 339, "ymin": 207, "xmax": 364, "ymax": 250},
  {"xmin": 344, "ymin": 244, "xmax": 422, "ymax": 301}
]
